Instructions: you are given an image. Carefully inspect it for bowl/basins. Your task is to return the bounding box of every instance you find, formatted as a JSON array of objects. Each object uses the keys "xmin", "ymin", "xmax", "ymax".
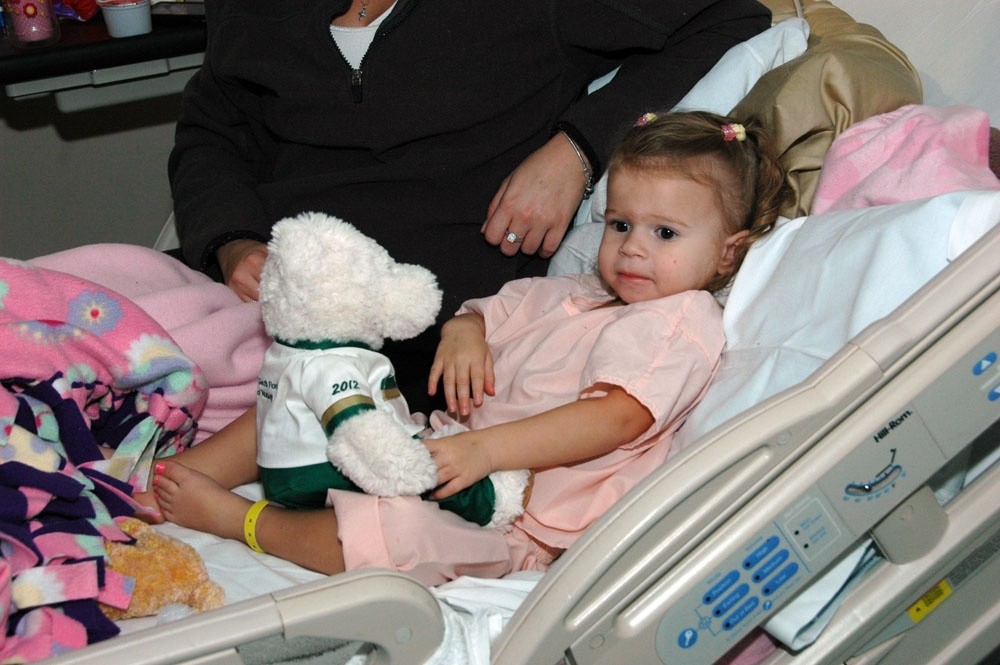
[{"xmin": 96, "ymin": 1, "xmax": 153, "ymax": 38}]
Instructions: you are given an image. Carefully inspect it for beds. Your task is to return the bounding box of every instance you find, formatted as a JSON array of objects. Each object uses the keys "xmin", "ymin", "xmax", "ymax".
[{"xmin": 18, "ymin": 189, "xmax": 1000, "ymax": 663}]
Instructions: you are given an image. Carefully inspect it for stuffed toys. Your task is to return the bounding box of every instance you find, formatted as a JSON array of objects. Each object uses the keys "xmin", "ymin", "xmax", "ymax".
[{"xmin": 255, "ymin": 210, "xmax": 535, "ymax": 533}]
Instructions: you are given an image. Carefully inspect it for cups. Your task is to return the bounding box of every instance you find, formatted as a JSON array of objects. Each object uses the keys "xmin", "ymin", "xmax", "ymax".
[{"xmin": 3, "ymin": 0, "xmax": 62, "ymax": 48}]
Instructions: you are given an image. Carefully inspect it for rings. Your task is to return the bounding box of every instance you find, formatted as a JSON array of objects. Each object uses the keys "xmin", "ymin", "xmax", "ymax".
[{"xmin": 505, "ymin": 229, "xmax": 525, "ymax": 244}]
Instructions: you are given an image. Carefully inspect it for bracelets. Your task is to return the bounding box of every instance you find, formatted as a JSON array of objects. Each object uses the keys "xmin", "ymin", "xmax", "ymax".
[{"xmin": 559, "ymin": 130, "xmax": 595, "ymax": 200}]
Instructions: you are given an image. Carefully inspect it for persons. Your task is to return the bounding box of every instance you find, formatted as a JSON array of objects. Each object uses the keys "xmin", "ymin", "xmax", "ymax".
[
  {"xmin": 160, "ymin": 1, "xmax": 775, "ymax": 429},
  {"xmin": 130, "ymin": 107, "xmax": 783, "ymax": 595}
]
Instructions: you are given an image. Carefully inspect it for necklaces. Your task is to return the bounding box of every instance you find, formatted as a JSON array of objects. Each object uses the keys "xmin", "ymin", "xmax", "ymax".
[{"xmin": 357, "ymin": 0, "xmax": 373, "ymax": 22}]
